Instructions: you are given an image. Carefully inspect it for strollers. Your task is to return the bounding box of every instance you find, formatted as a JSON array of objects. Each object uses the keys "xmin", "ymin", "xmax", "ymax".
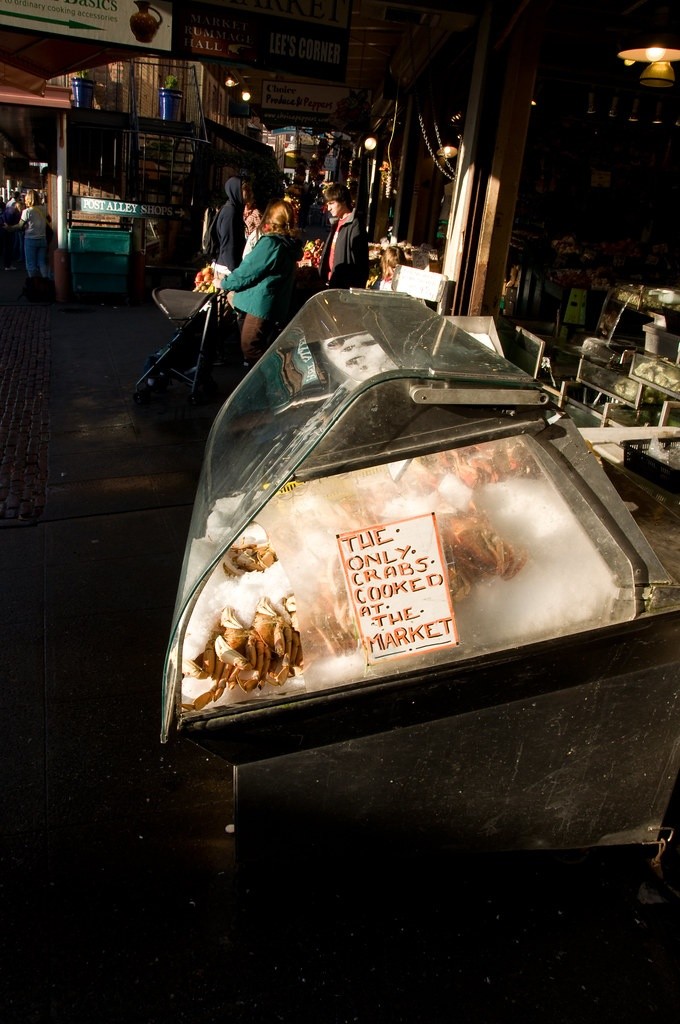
[{"xmin": 122, "ymin": 277, "xmax": 229, "ymax": 404}]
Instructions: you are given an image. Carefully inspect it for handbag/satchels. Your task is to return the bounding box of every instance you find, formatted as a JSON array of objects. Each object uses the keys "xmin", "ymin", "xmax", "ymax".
[{"xmin": 22, "ymin": 276, "xmax": 55, "ymax": 303}]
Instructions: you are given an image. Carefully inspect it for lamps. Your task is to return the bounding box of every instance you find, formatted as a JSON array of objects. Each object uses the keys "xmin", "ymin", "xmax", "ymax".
[
  {"xmin": 651, "ymin": 102, "xmax": 663, "ymax": 124},
  {"xmin": 587, "ymin": 92, "xmax": 596, "ymax": 115},
  {"xmin": 628, "ymin": 98, "xmax": 640, "ymax": 121},
  {"xmin": 608, "ymin": 97, "xmax": 619, "ymax": 117},
  {"xmin": 531, "ymin": 80, "xmax": 543, "ymax": 105},
  {"xmin": 616, "ymin": 33, "xmax": 680, "ymax": 89}
]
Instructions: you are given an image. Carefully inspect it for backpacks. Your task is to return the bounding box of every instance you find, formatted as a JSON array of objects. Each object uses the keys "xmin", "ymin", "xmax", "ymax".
[{"xmin": 201, "ymin": 205, "xmax": 223, "ymax": 255}]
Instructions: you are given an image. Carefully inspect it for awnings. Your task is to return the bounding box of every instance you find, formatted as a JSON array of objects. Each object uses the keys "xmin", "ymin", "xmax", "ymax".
[{"xmin": 206, "ymin": 118, "xmax": 280, "ymax": 170}]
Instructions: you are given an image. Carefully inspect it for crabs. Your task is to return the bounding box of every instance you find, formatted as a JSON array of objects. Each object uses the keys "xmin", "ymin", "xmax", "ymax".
[{"xmin": 177, "ymin": 442, "xmax": 543, "ymax": 712}]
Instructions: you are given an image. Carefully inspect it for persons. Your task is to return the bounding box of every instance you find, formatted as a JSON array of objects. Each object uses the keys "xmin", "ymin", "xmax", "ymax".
[
  {"xmin": 3, "ymin": 189, "xmax": 50, "ymax": 295},
  {"xmin": 379, "ymin": 246, "xmax": 407, "ymax": 291},
  {"xmin": 211, "ymin": 176, "xmax": 254, "ymax": 286},
  {"xmin": 315, "ymin": 183, "xmax": 369, "ymax": 290},
  {"xmin": 6, "ymin": 191, "xmax": 23, "ymax": 271},
  {"xmin": 211, "ymin": 200, "xmax": 305, "ymax": 365}
]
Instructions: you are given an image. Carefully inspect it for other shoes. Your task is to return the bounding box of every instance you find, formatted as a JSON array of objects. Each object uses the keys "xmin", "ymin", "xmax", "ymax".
[{"xmin": 5, "ymin": 266, "xmax": 16, "ymax": 270}]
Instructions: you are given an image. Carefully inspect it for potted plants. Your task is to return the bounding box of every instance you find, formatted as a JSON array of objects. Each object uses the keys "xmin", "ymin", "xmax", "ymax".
[
  {"xmin": 71, "ymin": 68, "xmax": 97, "ymax": 109},
  {"xmin": 157, "ymin": 75, "xmax": 184, "ymax": 122}
]
